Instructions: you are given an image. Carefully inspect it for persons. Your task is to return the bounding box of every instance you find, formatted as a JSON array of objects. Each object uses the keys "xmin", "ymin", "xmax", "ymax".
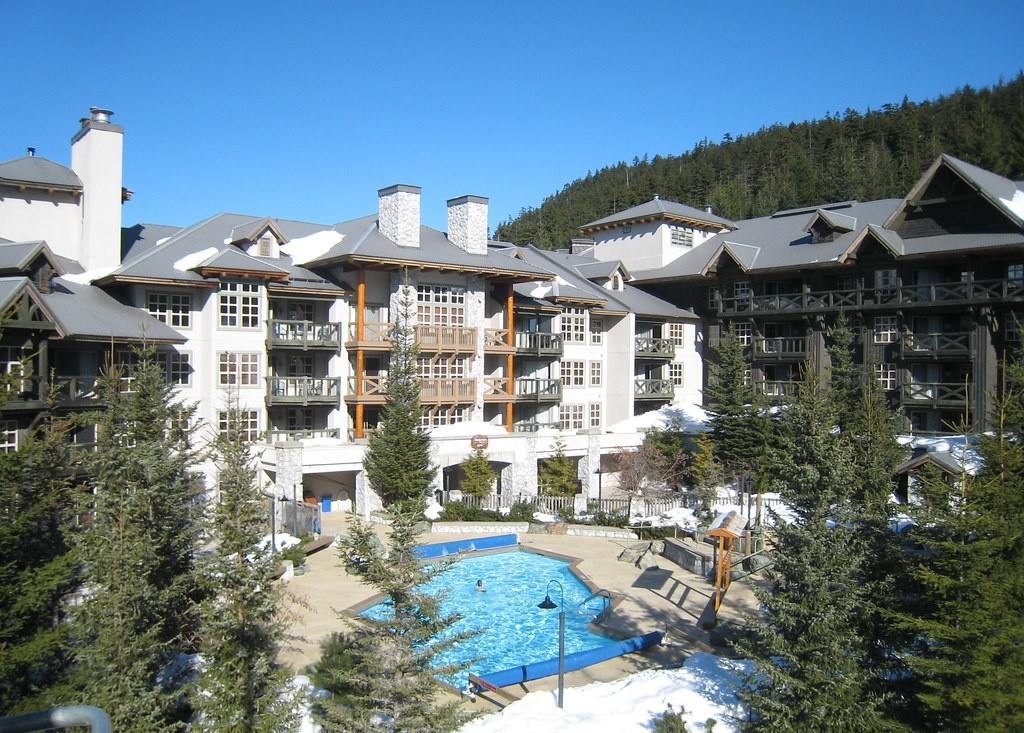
[{"xmin": 477, "ymin": 580, "xmax": 484, "ymax": 588}]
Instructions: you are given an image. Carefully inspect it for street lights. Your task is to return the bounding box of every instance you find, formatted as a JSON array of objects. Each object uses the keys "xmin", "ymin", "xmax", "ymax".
[
  {"xmin": 537, "ymin": 579, "xmax": 566, "ymax": 708},
  {"xmin": 271, "ymin": 484, "xmax": 290, "ymax": 554},
  {"xmin": 746, "ymin": 475, "xmax": 755, "ymax": 529},
  {"xmin": 594, "ymin": 468, "xmax": 602, "ymax": 513},
  {"xmin": 731, "ymin": 464, "xmax": 744, "ymax": 515}
]
